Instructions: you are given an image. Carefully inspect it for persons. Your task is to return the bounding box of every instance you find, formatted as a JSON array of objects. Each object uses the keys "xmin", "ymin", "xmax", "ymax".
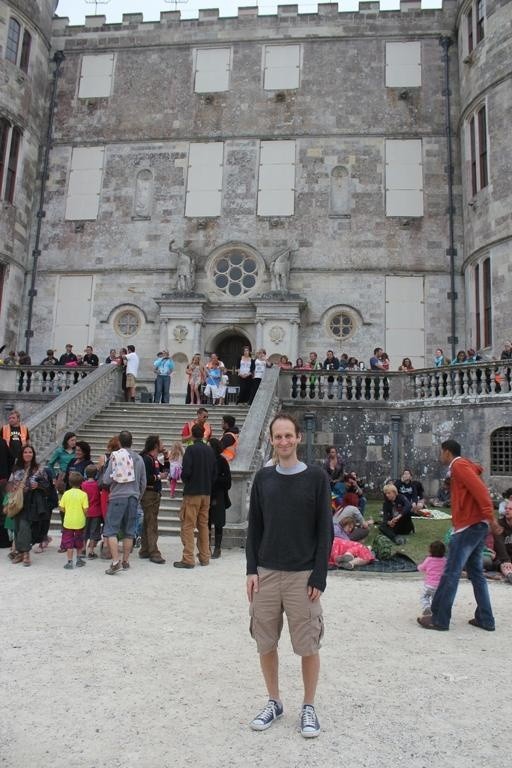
[
  {"xmin": 400, "ymin": 358, "xmax": 413, "ymax": 371},
  {"xmin": 185, "ymin": 353, "xmax": 228, "ymax": 405},
  {"xmin": 152, "ymin": 349, "xmax": 174, "ymax": 402},
  {"xmin": 418, "ymin": 540, "xmax": 450, "ymax": 614},
  {"xmin": 3, "ymin": 408, "xmax": 240, "ymax": 574},
  {"xmin": 433, "ymin": 344, "xmax": 512, "ymax": 396},
  {"xmin": 236, "ymin": 345, "xmax": 273, "ymax": 407},
  {"xmin": 168, "ymin": 239, "xmax": 198, "ymax": 295},
  {"xmin": 280, "ymin": 347, "xmax": 390, "ymax": 400},
  {"xmin": 417, "ymin": 438, "xmax": 501, "ymax": 631},
  {"xmin": 242, "ymin": 413, "xmax": 335, "ymax": 739},
  {"xmin": 107, "ymin": 345, "xmax": 139, "ymax": 402},
  {"xmin": 3, "ymin": 344, "xmax": 98, "ymax": 392}
]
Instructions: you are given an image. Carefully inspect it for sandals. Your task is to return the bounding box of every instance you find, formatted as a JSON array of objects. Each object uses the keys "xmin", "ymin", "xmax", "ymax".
[
  {"xmin": 469, "ymin": 619, "xmax": 495, "ymax": 631},
  {"xmin": 417, "ymin": 616, "xmax": 448, "ymax": 631}
]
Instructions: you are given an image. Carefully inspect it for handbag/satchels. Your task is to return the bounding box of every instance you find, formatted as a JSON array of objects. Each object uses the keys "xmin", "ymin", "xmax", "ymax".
[
  {"xmin": 373, "ymin": 534, "xmax": 392, "ymax": 560},
  {"xmin": 7, "ymin": 489, "xmax": 23, "ymax": 517}
]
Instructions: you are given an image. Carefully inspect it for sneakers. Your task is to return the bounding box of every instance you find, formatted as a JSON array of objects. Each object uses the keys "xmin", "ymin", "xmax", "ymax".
[
  {"xmin": 335, "ymin": 553, "xmax": 354, "ymax": 562},
  {"xmin": 396, "ymin": 537, "xmax": 406, "ymax": 545},
  {"xmin": 250, "ymin": 699, "xmax": 283, "ymax": 731},
  {"xmin": 336, "ymin": 562, "xmax": 354, "ymax": 569},
  {"xmin": 301, "ymin": 705, "xmax": 320, "ymax": 737},
  {"xmin": 7, "ymin": 537, "xmax": 221, "ymax": 575}
]
